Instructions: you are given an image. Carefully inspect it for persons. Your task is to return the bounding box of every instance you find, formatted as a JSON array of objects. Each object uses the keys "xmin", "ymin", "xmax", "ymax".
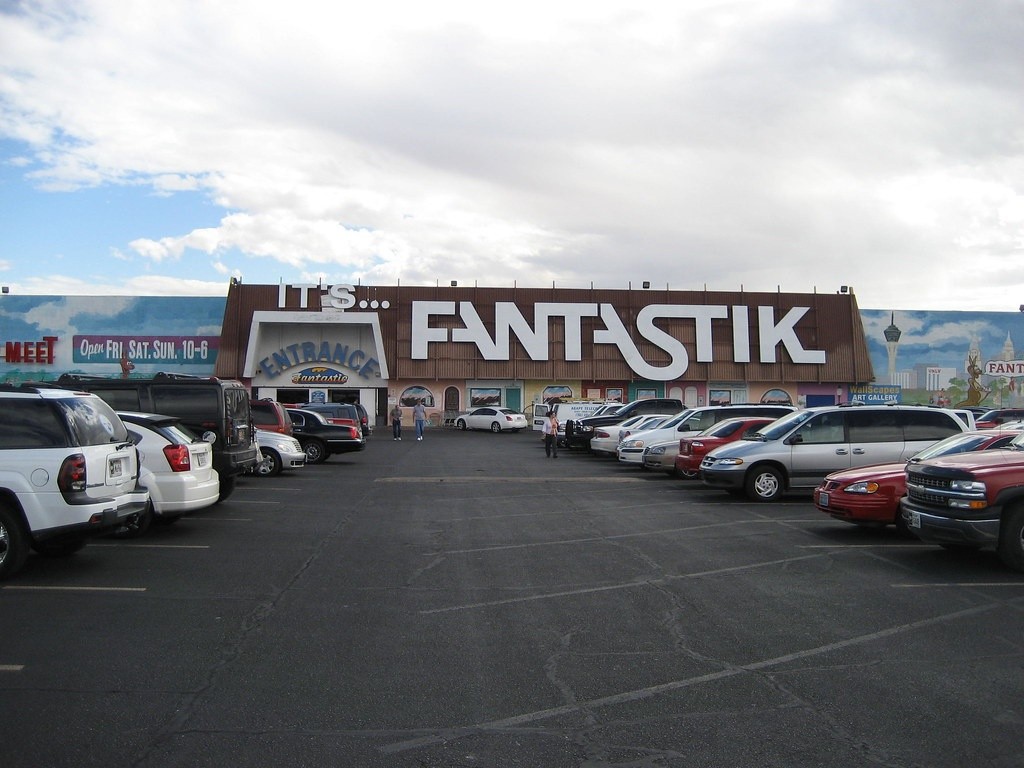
[
  {"xmin": 390, "ymin": 403, "xmax": 403, "ymax": 441},
  {"xmin": 542, "ymin": 411, "xmax": 559, "ymax": 458},
  {"xmin": 413, "ymin": 400, "xmax": 427, "ymax": 441}
]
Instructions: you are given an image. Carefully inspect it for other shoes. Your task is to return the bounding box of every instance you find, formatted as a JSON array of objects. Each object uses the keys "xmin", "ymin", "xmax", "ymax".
[
  {"xmin": 417, "ymin": 438, "xmax": 421, "ymax": 441},
  {"xmin": 394, "ymin": 438, "xmax": 397, "ymax": 441},
  {"xmin": 547, "ymin": 454, "xmax": 550, "ymax": 457},
  {"xmin": 420, "ymin": 436, "xmax": 423, "ymax": 440},
  {"xmin": 398, "ymin": 437, "xmax": 401, "ymax": 440},
  {"xmin": 553, "ymin": 455, "xmax": 558, "ymax": 458}
]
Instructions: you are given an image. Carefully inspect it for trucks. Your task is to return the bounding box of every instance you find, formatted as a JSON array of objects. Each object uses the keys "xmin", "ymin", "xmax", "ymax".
[{"xmin": 532, "ymin": 400, "xmax": 606, "ymax": 447}]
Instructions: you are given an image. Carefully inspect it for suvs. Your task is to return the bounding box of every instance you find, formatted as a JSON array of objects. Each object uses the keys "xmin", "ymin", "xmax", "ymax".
[
  {"xmin": 899, "ymin": 431, "xmax": 1024, "ymax": 571},
  {"xmin": 564, "ymin": 398, "xmax": 689, "ymax": 452},
  {"xmin": 55, "ymin": 371, "xmax": 259, "ymax": 502},
  {"xmin": 253, "ymin": 399, "xmax": 294, "ymax": 438},
  {"xmin": 114, "ymin": 410, "xmax": 220, "ymax": 538},
  {"xmin": 293, "ymin": 403, "xmax": 370, "ymax": 437},
  {"xmin": 0, "ymin": 382, "xmax": 151, "ymax": 576}
]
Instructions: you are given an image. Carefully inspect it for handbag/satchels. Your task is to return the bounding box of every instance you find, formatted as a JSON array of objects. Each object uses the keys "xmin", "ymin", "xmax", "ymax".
[{"xmin": 541, "ymin": 433, "xmax": 547, "ymax": 442}]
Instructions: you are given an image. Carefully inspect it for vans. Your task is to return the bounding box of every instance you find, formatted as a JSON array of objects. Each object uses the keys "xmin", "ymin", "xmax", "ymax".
[
  {"xmin": 616, "ymin": 404, "xmax": 799, "ymax": 467},
  {"xmin": 699, "ymin": 402, "xmax": 971, "ymax": 502}
]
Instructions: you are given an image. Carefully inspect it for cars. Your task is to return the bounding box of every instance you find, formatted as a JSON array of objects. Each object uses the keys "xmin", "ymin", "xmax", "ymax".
[
  {"xmin": 641, "ymin": 440, "xmax": 700, "ymax": 480},
  {"xmin": 250, "ymin": 426, "xmax": 308, "ymax": 476},
  {"xmin": 814, "ymin": 430, "xmax": 1024, "ymax": 533},
  {"xmin": 454, "ymin": 405, "xmax": 528, "ymax": 433},
  {"xmin": 588, "ymin": 412, "xmax": 674, "ymax": 456},
  {"xmin": 951, "ymin": 406, "xmax": 1024, "ymax": 430},
  {"xmin": 284, "ymin": 408, "xmax": 366, "ymax": 467},
  {"xmin": 679, "ymin": 416, "xmax": 777, "ymax": 473}
]
